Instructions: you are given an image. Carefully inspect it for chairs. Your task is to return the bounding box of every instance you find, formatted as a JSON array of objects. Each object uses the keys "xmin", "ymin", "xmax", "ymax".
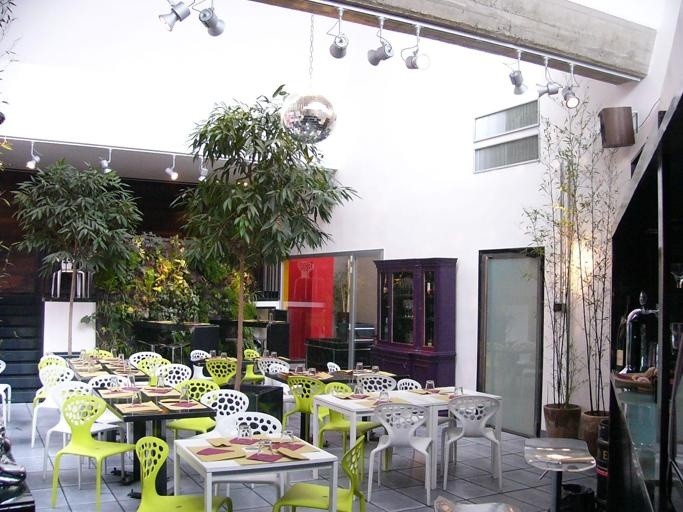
[{"xmin": 50, "ymin": 256, "xmax": 95, "ymax": 299}]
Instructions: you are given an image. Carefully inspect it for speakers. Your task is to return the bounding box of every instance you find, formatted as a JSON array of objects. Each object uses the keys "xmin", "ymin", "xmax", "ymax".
[{"xmin": 599, "ymin": 106, "xmax": 636, "ymax": 149}]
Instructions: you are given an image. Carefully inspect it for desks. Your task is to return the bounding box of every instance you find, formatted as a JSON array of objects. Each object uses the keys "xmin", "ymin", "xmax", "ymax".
[{"xmin": 522, "ymin": 438, "xmax": 597, "ymax": 512}]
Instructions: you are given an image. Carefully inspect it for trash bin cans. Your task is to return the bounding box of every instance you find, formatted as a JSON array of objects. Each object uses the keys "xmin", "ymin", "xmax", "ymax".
[{"xmin": 560, "ymin": 483, "xmax": 594, "ymax": 512}]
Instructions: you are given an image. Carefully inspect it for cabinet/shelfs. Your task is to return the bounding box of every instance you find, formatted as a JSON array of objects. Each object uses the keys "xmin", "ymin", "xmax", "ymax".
[{"xmin": 371, "ymin": 258, "xmax": 458, "ymax": 388}]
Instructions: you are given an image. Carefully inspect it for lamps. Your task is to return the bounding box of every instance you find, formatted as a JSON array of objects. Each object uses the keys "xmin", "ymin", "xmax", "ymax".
[
  {"xmin": 507, "ymin": 47, "xmax": 579, "ymax": 110},
  {"xmin": 25, "ymin": 141, "xmax": 40, "ymax": 171},
  {"xmin": 326, "ymin": 7, "xmax": 423, "ymax": 71},
  {"xmin": 157, "ymin": 0, "xmax": 224, "ymax": 37},
  {"xmin": 98, "ymin": 148, "xmax": 112, "ymax": 174},
  {"xmin": 164, "ymin": 153, "xmax": 179, "ymax": 182},
  {"xmin": 198, "ymin": 157, "xmax": 208, "ymax": 182}
]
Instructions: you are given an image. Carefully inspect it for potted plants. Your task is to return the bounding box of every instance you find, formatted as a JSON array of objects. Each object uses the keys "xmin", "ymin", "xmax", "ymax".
[
  {"xmin": 523, "ymin": 85, "xmax": 586, "ymax": 437},
  {"xmin": 170, "ymin": 85, "xmax": 362, "ymax": 430},
  {"xmin": 557, "ymin": 109, "xmax": 618, "ymax": 450},
  {"xmin": 7, "ymin": 159, "xmax": 142, "ymax": 358}
]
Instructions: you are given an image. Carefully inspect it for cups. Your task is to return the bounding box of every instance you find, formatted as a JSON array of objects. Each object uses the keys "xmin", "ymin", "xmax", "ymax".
[
  {"xmin": 281, "ymin": 431, "xmax": 294, "ymax": 444},
  {"xmin": 209, "ymin": 349, "xmax": 227, "ymax": 360},
  {"xmin": 356, "ymin": 362, "xmax": 380, "ymax": 375},
  {"xmin": 354, "ymin": 384, "xmax": 389, "ymax": 404},
  {"xmin": 133, "ymin": 391, "xmax": 142, "ymax": 408},
  {"xmin": 157, "ymin": 376, "xmax": 164, "ymax": 388},
  {"xmin": 425, "ymin": 380, "xmax": 463, "ymax": 397},
  {"xmin": 295, "ymin": 363, "xmax": 316, "ymax": 377},
  {"xmin": 126, "ymin": 375, "xmax": 136, "ymax": 389},
  {"xmin": 79, "ymin": 347, "xmax": 132, "ymax": 372},
  {"xmin": 263, "ymin": 350, "xmax": 277, "ymax": 359},
  {"xmin": 258, "ymin": 440, "xmax": 274, "ymax": 456},
  {"xmin": 238, "ymin": 425, "xmax": 254, "ymax": 443},
  {"xmin": 180, "ymin": 387, "xmax": 189, "ymax": 404}
]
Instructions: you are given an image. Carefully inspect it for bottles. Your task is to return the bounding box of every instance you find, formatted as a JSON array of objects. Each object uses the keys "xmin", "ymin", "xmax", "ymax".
[
  {"xmin": 426, "ymin": 272, "xmax": 434, "ymax": 296},
  {"xmin": 427, "ymin": 328, "xmax": 433, "ymax": 346},
  {"xmin": 269, "ymin": 313, "xmax": 273, "ymax": 322},
  {"xmin": 383, "ymin": 306, "xmax": 388, "ymax": 341},
  {"xmin": 383, "ymin": 273, "xmax": 388, "ymax": 294},
  {"xmin": 194, "ymin": 314, "xmax": 199, "ymax": 324},
  {"xmin": 403, "ymin": 300, "xmax": 414, "ymax": 319}
]
